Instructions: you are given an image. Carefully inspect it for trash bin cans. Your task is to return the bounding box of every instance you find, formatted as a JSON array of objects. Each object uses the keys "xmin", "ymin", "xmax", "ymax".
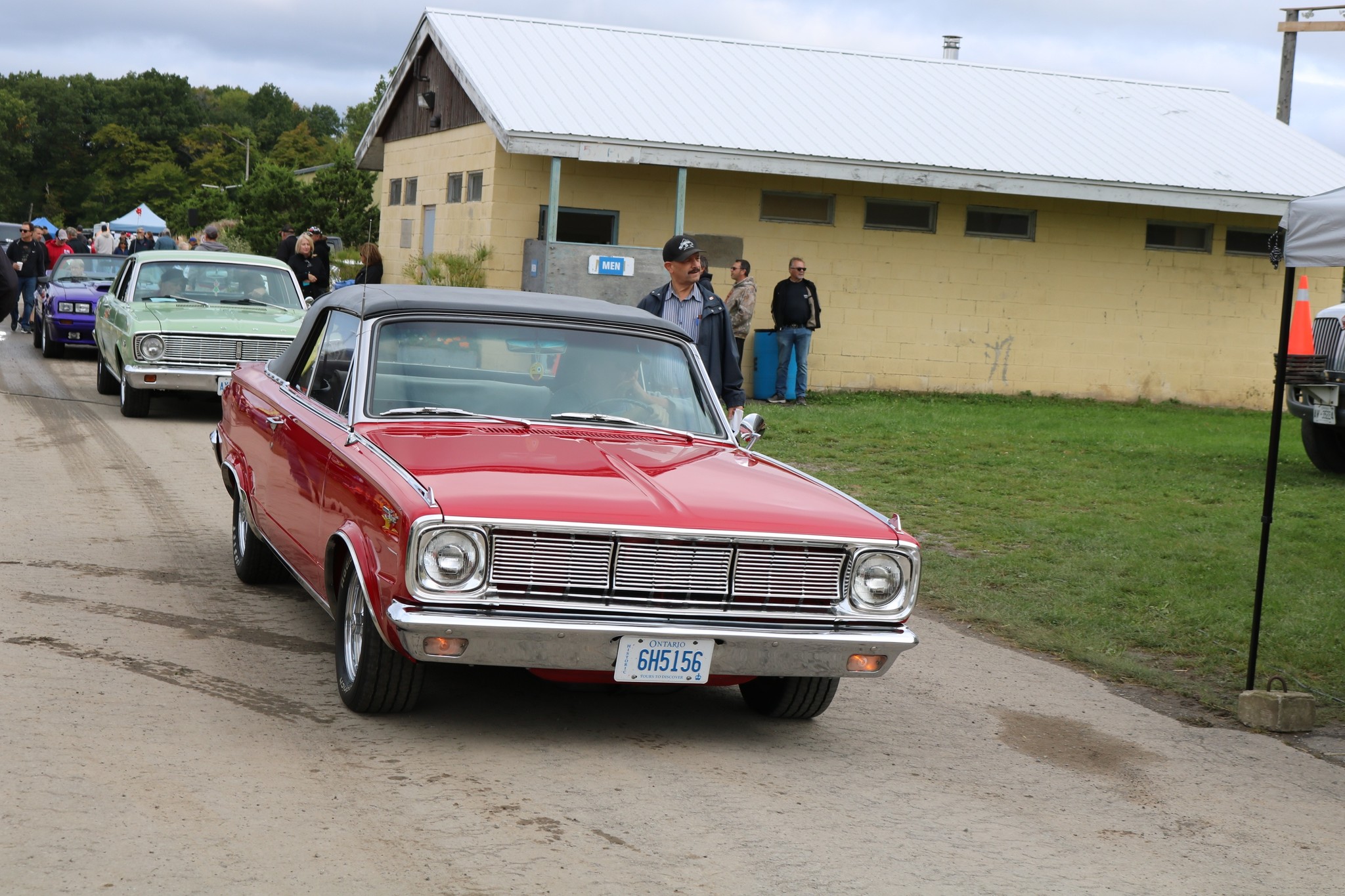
[{"xmin": 752, "ymin": 327, "xmax": 798, "ymax": 400}]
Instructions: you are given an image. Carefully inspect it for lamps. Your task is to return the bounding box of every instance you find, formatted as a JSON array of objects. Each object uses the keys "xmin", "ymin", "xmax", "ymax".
[{"xmin": 416, "ymin": 91, "xmax": 435, "ymax": 110}]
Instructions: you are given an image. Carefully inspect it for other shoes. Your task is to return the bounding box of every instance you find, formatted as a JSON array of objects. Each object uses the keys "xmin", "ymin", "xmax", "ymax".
[
  {"xmin": 20, "ymin": 324, "xmax": 32, "ymax": 333},
  {"xmin": 11, "ymin": 314, "xmax": 19, "ymax": 331},
  {"xmin": 18, "ymin": 317, "xmax": 34, "ymax": 331}
]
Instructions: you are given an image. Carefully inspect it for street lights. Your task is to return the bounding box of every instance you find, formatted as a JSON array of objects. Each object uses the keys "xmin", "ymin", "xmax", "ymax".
[{"xmin": 202, "ymin": 125, "xmax": 249, "ymax": 181}]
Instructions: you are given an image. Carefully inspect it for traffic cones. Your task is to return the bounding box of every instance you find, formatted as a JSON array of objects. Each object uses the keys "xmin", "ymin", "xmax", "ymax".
[{"xmin": 1273, "ymin": 275, "xmax": 1327, "ymax": 384}]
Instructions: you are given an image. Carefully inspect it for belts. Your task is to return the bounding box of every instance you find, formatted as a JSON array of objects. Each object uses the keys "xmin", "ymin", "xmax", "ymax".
[{"xmin": 783, "ymin": 323, "xmax": 806, "ymax": 329}]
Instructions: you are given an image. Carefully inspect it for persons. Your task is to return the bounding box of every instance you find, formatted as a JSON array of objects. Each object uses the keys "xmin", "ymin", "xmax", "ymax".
[
  {"xmin": 698, "ymin": 255, "xmax": 715, "ymax": 293},
  {"xmin": 355, "ymin": 242, "xmax": 383, "ymax": 284},
  {"xmin": 636, "ymin": 234, "xmax": 747, "ymax": 420},
  {"xmin": 148, "ymin": 268, "xmax": 190, "ymax": 299},
  {"xmin": 0, "ymin": 224, "xmax": 229, "ymax": 334},
  {"xmin": 238, "ymin": 272, "xmax": 277, "ymax": 305},
  {"xmin": 722, "ymin": 259, "xmax": 757, "ymax": 373},
  {"xmin": 276, "ymin": 223, "xmax": 330, "ymax": 300},
  {"xmin": 766, "ymin": 258, "xmax": 821, "ymax": 406},
  {"xmin": 541, "ymin": 335, "xmax": 677, "ymax": 428}
]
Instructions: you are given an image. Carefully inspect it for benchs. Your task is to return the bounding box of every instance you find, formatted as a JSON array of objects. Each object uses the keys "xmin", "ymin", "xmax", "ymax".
[{"xmin": 327, "ymin": 366, "xmax": 551, "ymax": 417}]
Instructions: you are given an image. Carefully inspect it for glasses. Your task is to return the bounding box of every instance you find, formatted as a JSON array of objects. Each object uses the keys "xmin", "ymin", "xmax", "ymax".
[
  {"xmin": 360, "ymin": 253, "xmax": 363, "ymax": 256},
  {"xmin": 731, "ymin": 267, "xmax": 744, "ymax": 270},
  {"xmin": 137, "ymin": 232, "xmax": 142, "ymax": 234},
  {"xmin": 791, "ymin": 268, "xmax": 806, "ymax": 271},
  {"xmin": 121, "ymin": 243, "xmax": 126, "ymax": 245},
  {"xmin": 19, "ymin": 229, "xmax": 31, "ymax": 233},
  {"xmin": 253, "ymin": 282, "xmax": 265, "ymax": 286}
]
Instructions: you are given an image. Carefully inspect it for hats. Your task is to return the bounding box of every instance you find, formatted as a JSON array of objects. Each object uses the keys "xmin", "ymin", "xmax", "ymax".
[
  {"xmin": 162, "ymin": 228, "xmax": 170, "ymax": 235},
  {"xmin": 595, "ymin": 337, "xmax": 650, "ymax": 364},
  {"xmin": 205, "ymin": 225, "xmax": 218, "ymax": 238},
  {"xmin": 55, "ymin": 228, "xmax": 68, "ymax": 240},
  {"xmin": 161, "ymin": 268, "xmax": 189, "ymax": 284},
  {"xmin": 188, "ymin": 237, "xmax": 197, "ymax": 242},
  {"xmin": 120, "ymin": 231, "xmax": 125, "ymax": 235},
  {"xmin": 662, "ymin": 235, "xmax": 707, "ymax": 264},
  {"xmin": 306, "ymin": 225, "xmax": 323, "ymax": 236},
  {"xmin": 278, "ymin": 224, "xmax": 295, "ymax": 235}
]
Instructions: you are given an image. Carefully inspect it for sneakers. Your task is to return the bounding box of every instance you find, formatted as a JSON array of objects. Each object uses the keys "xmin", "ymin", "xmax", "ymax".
[
  {"xmin": 795, "ymin": 396, "xmax": 807, "ymax": 405},
  {"xmin": 767, "ymin": 393, "xmax": 786, "ymax": 403}
]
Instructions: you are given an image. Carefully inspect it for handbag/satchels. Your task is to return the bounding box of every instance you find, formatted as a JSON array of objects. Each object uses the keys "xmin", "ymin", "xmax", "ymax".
[{"xmin": 729, "ymin": 408, "xmax": 744, "ymax": 443}]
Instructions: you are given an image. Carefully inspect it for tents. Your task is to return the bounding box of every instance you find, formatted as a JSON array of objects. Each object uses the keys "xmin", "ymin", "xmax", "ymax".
[
  {"xmin": 1243, "ymin": 185, "xmax": 1345, "ymax": 692},
  {"xmin": 34, "ymin": 217, "xmax": 59, "ymax": 240},
  {"xmin": 108, "ymin": 203, "xmax": 167, "ymax": 234}
]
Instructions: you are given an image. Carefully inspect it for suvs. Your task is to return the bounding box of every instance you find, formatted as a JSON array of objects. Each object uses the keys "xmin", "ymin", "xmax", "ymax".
[{"xmin": 1287, "ymin": 302, "xmax": 1345, "ymax": 473}]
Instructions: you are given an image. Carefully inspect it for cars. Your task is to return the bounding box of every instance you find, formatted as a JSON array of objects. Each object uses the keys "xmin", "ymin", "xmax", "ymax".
[
  {"xmin": 209, "ymin": 284, "xmax": 919, "ymax": 720},
  {"xmin": 33, "ymin": 253, "xmax": 131, "ymax": 359},
  {"xmin": 91, "ymin": 250, "xmax": 322, "ymax": 419},
  {"xmin": 0, "ymin": 222, "xmax": 46, "ymax": 254}
]
